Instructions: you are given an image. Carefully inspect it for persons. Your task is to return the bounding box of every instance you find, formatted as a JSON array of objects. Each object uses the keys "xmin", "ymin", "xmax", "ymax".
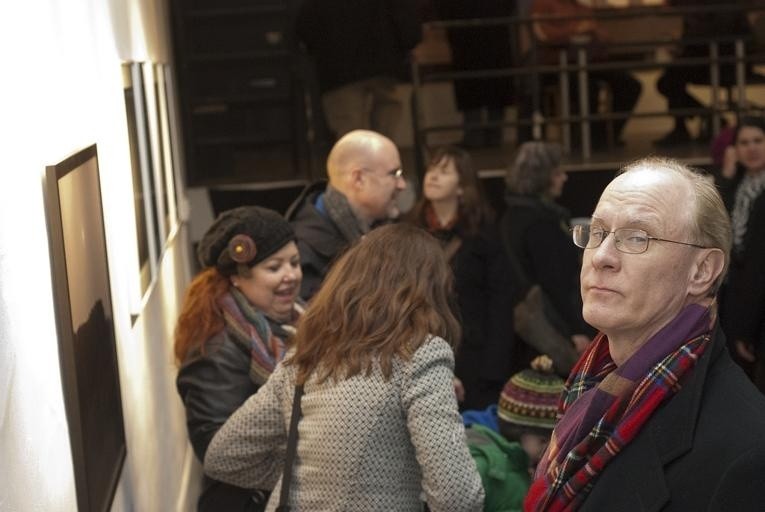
[
  {"xmin": 200, "ymin": 222, "xmax": 485, "ymax": 512},
  {"xmin": 499, "ymin": 138, "xmax": 595, "ymax": 370},
  {"xmin": 528, "ymin": 0, "xmax": 642, "ymax": 150},
  {"xmin": 716, "ymin": 115, "xmax": 765, "ymax": 394},
  {"xmin": 468, "ymin": 356, "xmax": 565, "ymax": 512},
  {"xmin": 411, "ymin": 144, "xmax": 499, "ymax": 412},
  {"xmin": 175, "ymin": 206, "xmax": 310, "ymax": 512},
  {"xmin": 286, "ymin": 128, "xmax": 407, "ymax": 302},
  {"xmin": 521, "ymin": 157, "xmax": 764, "ymax": 512},
  {"xmin": 446, "ymin": 0, "xmax": 521, "ymax": 149},
  {"xmin": 652, "ymin": 0, "xmax": 747, "ymax": 149}
]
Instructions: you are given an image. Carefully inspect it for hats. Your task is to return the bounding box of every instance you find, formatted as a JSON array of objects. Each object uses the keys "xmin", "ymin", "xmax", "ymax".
[
  {"xmin": 196, "ymin": 206, "xmax": 294, "ymax": 274},
  {"xmin": 497, "ymin": 354, "xmax": 566, "ymax": 434}
]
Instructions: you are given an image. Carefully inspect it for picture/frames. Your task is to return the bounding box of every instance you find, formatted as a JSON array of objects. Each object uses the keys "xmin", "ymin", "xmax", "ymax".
[
  {"xmin": 145, "ymin": 62, "xmax": 181, "ymax": 248},
  {"xmin": 120, "ymin": 62, "xmax": 155, "ymax": 318},
  {"xmin": 45, "ymin": 143, "xmax": 127, "ymax": 511}
]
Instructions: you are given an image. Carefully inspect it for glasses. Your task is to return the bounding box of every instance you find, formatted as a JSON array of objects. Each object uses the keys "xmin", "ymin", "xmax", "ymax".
[
  {"xmin": 572, "ymin": 222, "xmax": 720, "ymax": 256},
  {"xmin": 355, "ymin": 167, "xmax": 404, "ymax": 184}
]
